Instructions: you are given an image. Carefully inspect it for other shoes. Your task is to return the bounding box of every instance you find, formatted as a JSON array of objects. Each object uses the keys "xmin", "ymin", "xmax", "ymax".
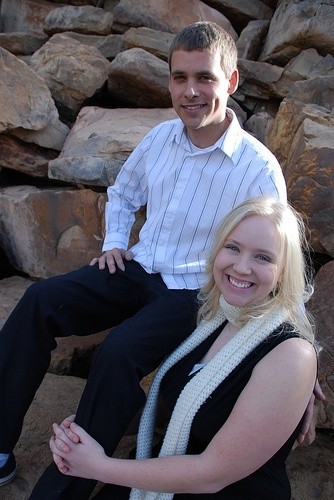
[{"xmin": 0, "ymin": 451, "xmax": 18, "ymax": 488}]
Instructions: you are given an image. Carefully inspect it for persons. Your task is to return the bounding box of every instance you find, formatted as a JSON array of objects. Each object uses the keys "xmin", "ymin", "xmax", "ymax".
[
  {"xmin": 49, "ymin": 195, "xmax": 319, "ymax": 500},
  {"xmin": 0, "ymin": 20, "xmax": 328, "ymax": 500}
]
